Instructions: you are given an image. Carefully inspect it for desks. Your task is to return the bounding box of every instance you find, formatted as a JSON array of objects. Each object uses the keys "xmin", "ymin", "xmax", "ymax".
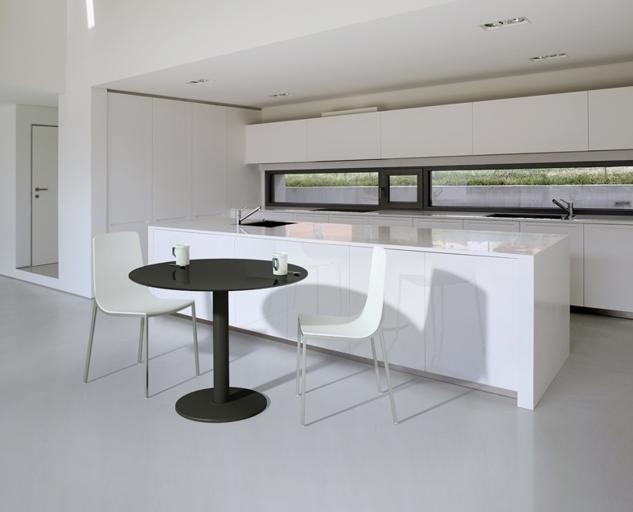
[{"xmin": 128, "ymin": 256, "xmax": 308, "ymax": 423}]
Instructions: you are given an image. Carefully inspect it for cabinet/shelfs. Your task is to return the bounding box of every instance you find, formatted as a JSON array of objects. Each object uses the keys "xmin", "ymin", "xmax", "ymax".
[{"xmin": 241, "ymin": 85, "xmax": 633, "ymax": 164}]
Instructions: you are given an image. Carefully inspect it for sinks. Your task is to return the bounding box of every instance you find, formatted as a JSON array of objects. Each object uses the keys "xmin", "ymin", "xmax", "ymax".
[
  {"xmin": 231, "ymin": 218, "xmax": 297, "ymax": 227},
  {"xmin": 485, "ymin": 213, "xmax": 576, "ymax": 220}
]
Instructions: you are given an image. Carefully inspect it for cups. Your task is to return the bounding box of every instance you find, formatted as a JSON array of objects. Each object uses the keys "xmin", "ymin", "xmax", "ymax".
[
  {"xmin": 172, "ymin": 245, "xmax": 191, "ymax": 267},
  {"xmin": 271, "ymin": 254, "xmax": 289, "ymax": 276}
]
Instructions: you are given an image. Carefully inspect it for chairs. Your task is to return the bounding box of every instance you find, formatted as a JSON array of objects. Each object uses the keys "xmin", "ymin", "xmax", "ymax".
[
  {"xmin": 83, "ymin": 230, "xmax": 200, "ymax": 399},
  {"xmin": 293, "ymin": 241, "xmax": 394, "ymax": 401}
]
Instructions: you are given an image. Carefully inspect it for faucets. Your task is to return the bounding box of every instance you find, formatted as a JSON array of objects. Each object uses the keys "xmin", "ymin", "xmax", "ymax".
[
  {"xmin": 235, "ymin": 224, "xmax": 250, "ymax": 235},
  {"xmin": 234, "ymin": 205, "xmax": 261, "ymax": 224},
  {"xmin": 553, "ymin": 198, "xmax": 573, "ymax": 217}
]
